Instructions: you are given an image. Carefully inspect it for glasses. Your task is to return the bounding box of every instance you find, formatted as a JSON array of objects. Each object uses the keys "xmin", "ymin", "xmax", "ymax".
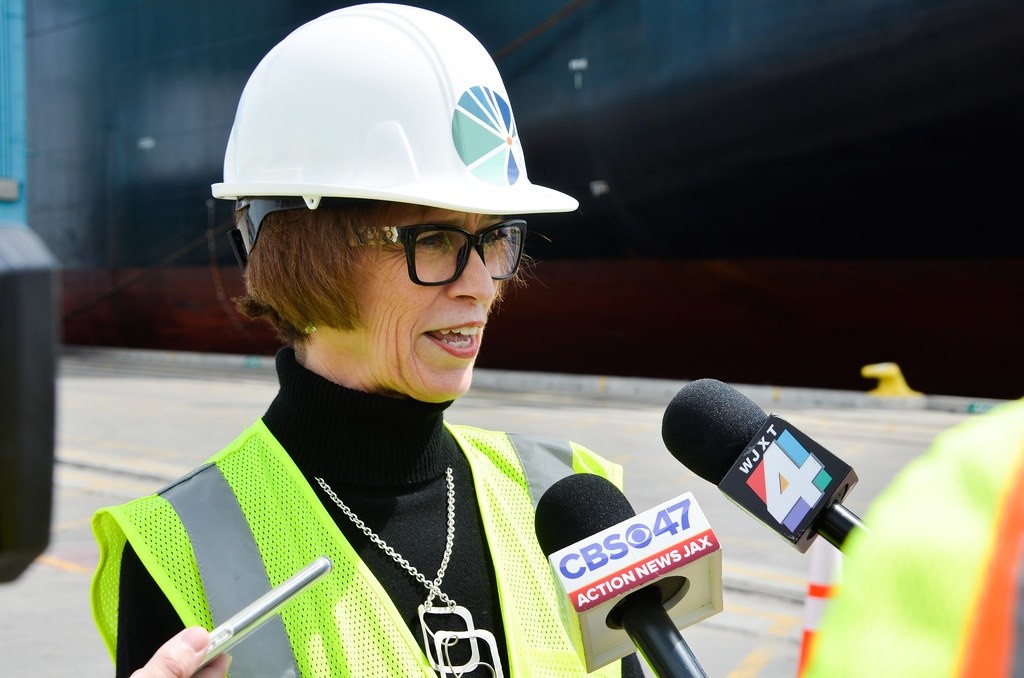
[{"xmin": 356, "ymin": 219, "xmax": 528, "ymax": 287}]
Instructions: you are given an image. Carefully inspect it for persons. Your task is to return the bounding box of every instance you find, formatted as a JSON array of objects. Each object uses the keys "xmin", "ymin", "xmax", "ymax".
[
  {"xmin": 803, "ymin": 394, "xmax": 1024, "ymax": 678},
  {"xmin": 123, "ymin": 625, "xmax": 233, "ymax": 678},
  {"xmin": 91, "ymin": 3, "xmax": 654, "ymax": 678}
]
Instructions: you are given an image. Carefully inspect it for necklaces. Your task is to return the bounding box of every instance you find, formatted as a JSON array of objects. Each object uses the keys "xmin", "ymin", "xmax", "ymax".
[{"xmin": 315, "ymin": 464, "xmax": 505, "ymax": 678}]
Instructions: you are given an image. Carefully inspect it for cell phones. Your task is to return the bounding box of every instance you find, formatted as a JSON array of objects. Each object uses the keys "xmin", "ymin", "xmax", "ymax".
[{"xmin": 193, "ymin": 556, "xmax": 331, "ymax": 673}]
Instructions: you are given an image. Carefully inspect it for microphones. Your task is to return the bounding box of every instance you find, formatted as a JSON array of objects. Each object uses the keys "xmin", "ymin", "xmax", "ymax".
[
  {"xmin": 661, "ymin": 379, "xmax": 870, "ymax": 554},
  {"xmin": 535, "ymin": 472, "xmax": 723, "ymax": 678}
]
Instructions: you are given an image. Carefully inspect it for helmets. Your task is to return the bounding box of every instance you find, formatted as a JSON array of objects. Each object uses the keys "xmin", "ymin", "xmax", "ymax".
[{"xmin": 211, "ymin": 2, "xmax": 581, "ymax": 217}]
